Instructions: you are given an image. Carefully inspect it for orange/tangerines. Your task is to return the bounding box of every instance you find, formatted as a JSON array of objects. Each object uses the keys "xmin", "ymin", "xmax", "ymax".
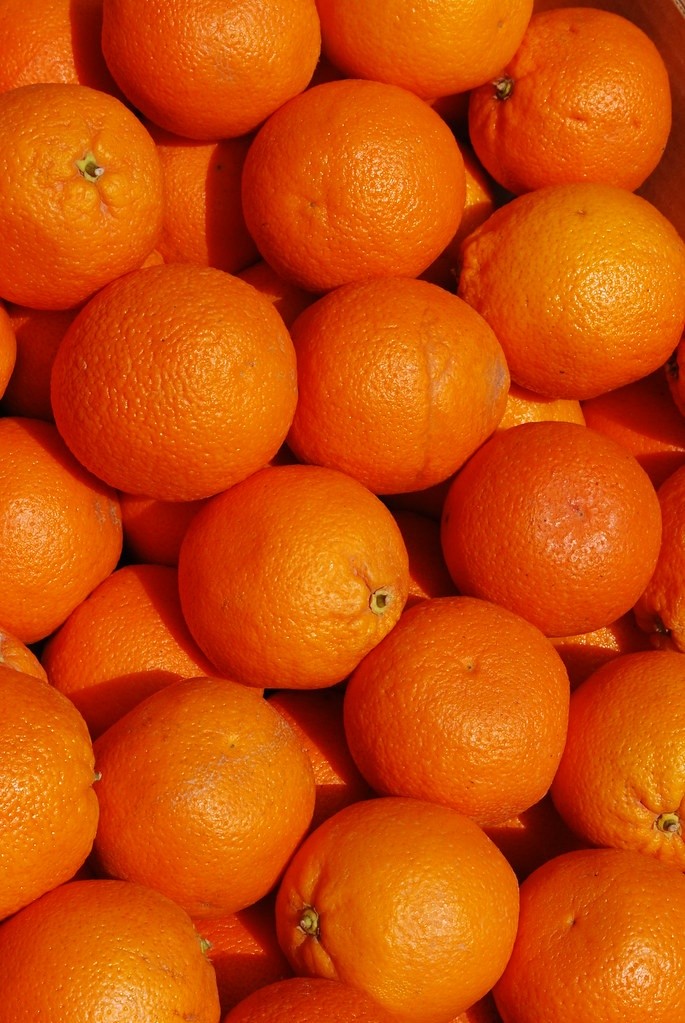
[{"xmin": 0, "ymin": 0, "xmax": 685, "ymax": 1023}]
